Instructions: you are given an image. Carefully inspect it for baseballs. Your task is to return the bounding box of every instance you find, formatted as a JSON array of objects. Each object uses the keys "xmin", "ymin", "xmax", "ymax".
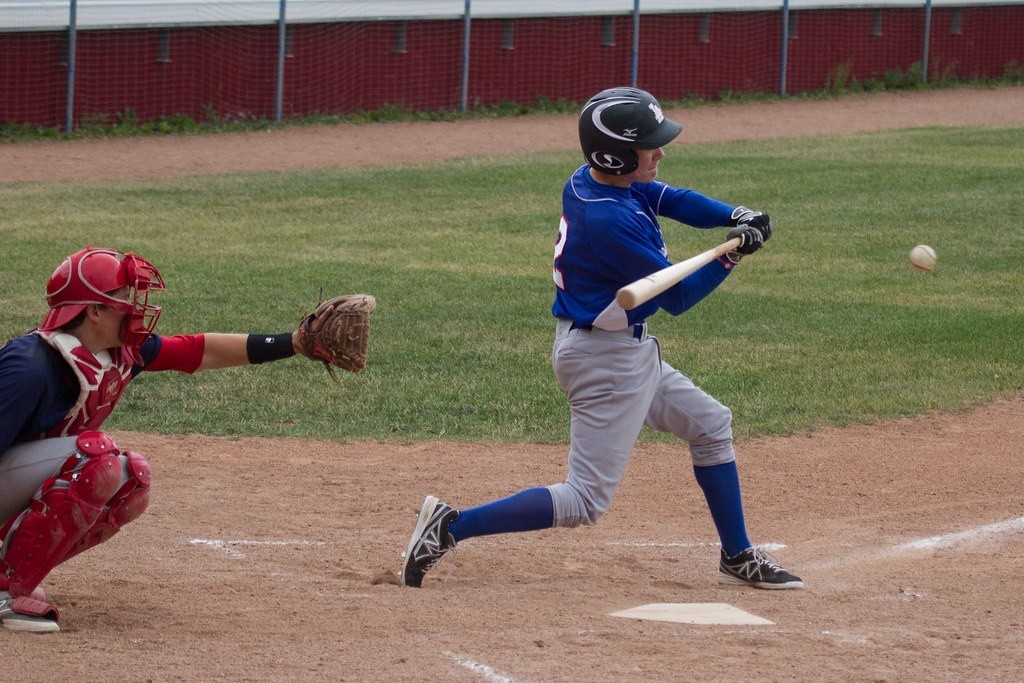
[{"xmin": 908, "ymin": 245, "xmax": 938, "ymax": 271}]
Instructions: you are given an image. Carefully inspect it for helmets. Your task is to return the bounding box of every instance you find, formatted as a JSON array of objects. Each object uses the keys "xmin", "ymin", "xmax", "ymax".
[
  {"xmin": 578, "ymin": 85, "xmax": 683, "ymax": 175},
  {"xmin": 38, "ymin": 246, "xmax": 129, "ymax": 331}
]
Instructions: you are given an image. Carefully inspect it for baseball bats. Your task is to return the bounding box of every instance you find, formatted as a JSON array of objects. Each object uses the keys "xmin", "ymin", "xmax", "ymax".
[{"xmin": 615, "ymin": 236, "xmax": 745, "ymax": 310}]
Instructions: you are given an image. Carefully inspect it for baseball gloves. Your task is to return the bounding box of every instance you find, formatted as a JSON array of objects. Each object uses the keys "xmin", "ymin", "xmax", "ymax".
[{"xmin": 298, "ymin": 286, "xmax": 376, "ymax": 383}]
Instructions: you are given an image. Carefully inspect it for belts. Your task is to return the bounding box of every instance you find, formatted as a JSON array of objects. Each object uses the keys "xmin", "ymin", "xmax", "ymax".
[{"xmin": 568, "ymin": 320, "xmax": 643, "ymax": 338}]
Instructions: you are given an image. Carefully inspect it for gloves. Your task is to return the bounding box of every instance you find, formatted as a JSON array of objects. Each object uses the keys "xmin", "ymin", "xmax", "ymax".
[{"xmin": 722, "ymin": 207, "xmax": 773, "ymax": 265}]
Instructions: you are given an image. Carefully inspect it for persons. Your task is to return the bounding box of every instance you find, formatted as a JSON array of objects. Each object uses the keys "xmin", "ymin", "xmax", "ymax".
[
  {"xmin": 400, "ymin": 87, "xmax": 803, "ymax": 588},
  {"xmin": 0, "ymin": 246, "xmax": 333, "ymax": 631}
]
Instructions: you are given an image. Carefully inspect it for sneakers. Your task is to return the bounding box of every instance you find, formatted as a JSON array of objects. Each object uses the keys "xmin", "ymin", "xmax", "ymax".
[
  {"xmin": 0, "ymin": 590, "xmax": 61, "ymax": 635},
  {"xmin": 715, "ymin": 548, "xmax": 804, "ymax": 591},
  {"xmin": 402, "ymin": 496, "xmax": 461, "ymax": 589}
]
